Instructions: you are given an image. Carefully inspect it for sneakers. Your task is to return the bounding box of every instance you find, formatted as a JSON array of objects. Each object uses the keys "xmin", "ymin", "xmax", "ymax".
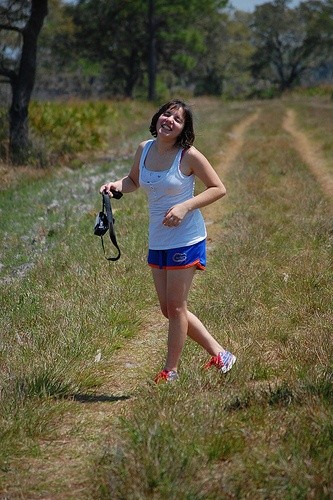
[
  {"xmin": 203, "ymin": 351, "xmax": 238, "ymax": 374},
  {"xmin": 153, "ymin": 370, "xmax": 181, "ymax": 385}
]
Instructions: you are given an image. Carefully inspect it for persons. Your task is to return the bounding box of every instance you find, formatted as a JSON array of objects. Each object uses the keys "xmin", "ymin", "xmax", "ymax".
[{"xmin": 99, "ymin": 99, "xmax": 237, "ymax": 384}]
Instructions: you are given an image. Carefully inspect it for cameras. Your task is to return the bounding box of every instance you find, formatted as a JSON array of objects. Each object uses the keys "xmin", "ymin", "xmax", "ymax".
[{"xmin": 94, "ymin": 212, "xmax": 115, "ymax": 236}]
